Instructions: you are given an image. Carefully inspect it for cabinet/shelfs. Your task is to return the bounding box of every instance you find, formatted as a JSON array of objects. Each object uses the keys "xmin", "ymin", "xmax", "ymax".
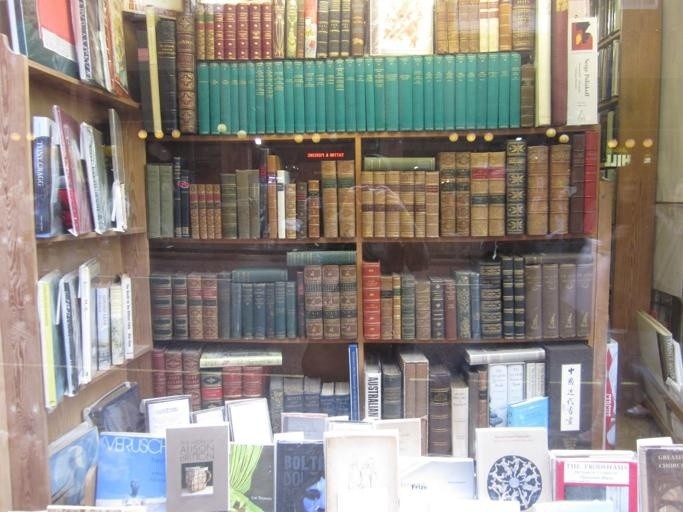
[
  {"xmin": 595, "ymin": 1, "xmax": 665, "ymax": 386},
  {"xmin": 3, "ymin": 36, "xmax": 155, "ymax": 509},
  {"xmin": 153, "ymin": 132, "xmax": 360, "ymax": 417},
  {"xmin": 358, "ymin": 125, "xmax": 602, "ymax": 450}
]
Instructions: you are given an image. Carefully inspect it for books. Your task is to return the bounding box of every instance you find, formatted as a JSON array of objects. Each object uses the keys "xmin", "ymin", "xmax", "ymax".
[{"xmin": 0, "ymin": 0, "xmax": 681, "ymax": 512}]
